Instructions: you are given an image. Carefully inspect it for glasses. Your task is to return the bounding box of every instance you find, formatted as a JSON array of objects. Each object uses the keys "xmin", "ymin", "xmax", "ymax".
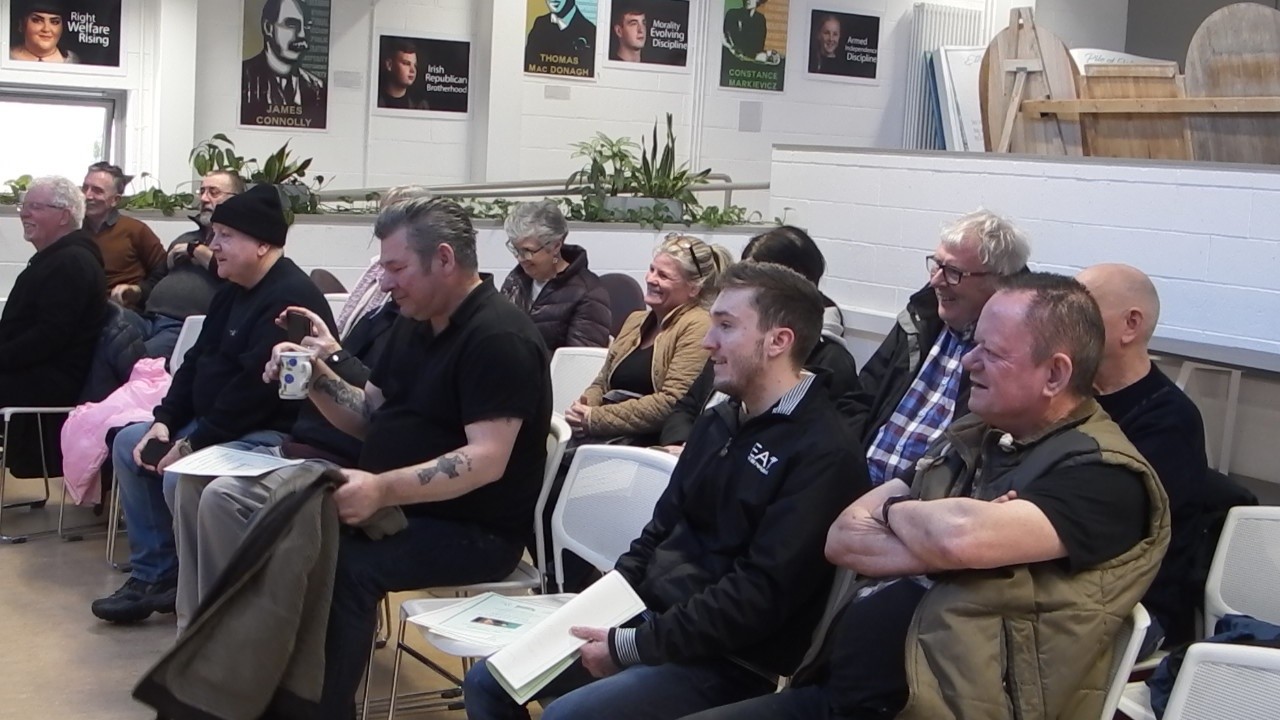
[
  {"xmin": 505, "ymin": 239, "xmax": 550, "ymax": 260},
  {"xmin": 664, "ymin": 230, "xmax": 703, "ymax": 278},
  {"xmin": 925, "ymin": 254, "xmax": 996, "ymax": 287},
  {"xmin": 16, "ymin": 202, "xmax": 67, "ymax": 214},
  {"xmin": 195, "ymin": 188, "xmax": 237, "ymax": 200}
]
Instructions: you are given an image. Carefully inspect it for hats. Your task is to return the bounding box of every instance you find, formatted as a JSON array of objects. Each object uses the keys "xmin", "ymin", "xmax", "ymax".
[{"xmin": 209, "ymin": 182, "xmax": 288, "ymax": 248}]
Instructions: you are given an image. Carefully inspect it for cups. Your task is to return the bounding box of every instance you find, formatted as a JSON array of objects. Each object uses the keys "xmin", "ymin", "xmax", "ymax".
[{"xmin": 278, "ymin": 352, "xmax": 313, "ymax": 400}]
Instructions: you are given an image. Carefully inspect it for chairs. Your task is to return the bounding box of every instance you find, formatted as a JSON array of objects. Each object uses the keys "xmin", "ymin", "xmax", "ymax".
[
  {"xmin": 359, "ymin": 418, "xmax": 683, "ymax": 720},
  {"xmin": 55, "ymin": 314, "xmax": 208, "ymax": 565},
  {"xmin": 0, "ymin": 400, "xmax": 78, "ymax": 541},
  {"xmin": 551, "ymin": 344, "xmax": 609, "ymax": 412},
  {"xmin": 1099, "ymin": 502, "xmax": 1280, "ymax": 720}
]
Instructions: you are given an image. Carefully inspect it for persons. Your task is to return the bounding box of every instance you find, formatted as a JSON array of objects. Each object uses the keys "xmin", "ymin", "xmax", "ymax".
[
  {"xmin": 9, "ymin": 0, "xmax": 82, "ymax": 66},
  {"xmin": 523, "ymin": 0, "xmax": 597, "ymax": 78},
  {"xmin": 238, "ymin": 0, "xmax": 331, "ymax": 128},
  {"xmin": 499, "ymin": 201, "xmax": 611, "ymax": 348},
  {"xmin": 263, "ymin": 195, "xmax": 554, "ymax": 720},
  {"xmin": 723, "ymin": 0, "xmax": 780, "ymax": 68},
  {"xmin": 811, "ymin": 14, "xmax": 842, "ymax": 74},
  {"xmin": 610, "ymin": 9, "xmax": 647, "ymax": 62},
  {"xmin": 563, "ymin": 210, "xmax": 1207, "ymax": 720},
  {"xmin": 377, "ymin": 36, "xmax": 430, "ymax": 110},
  {"xmin": 0, "ymin": 161, "xmax": 432, "ymax": 720},
  {"xmin": 658, "ymin": 225, "xmax": 868, "ymax": 447}
]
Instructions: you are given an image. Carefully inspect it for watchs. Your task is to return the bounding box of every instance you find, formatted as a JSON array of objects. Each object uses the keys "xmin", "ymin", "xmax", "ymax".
[
  {"xmin": 187, "ymin": 238, "xmax": 202, "ymax": 257},
  {"xmin": 881, "ymin": 494, "xmax": 915, "ymax": 527},
  {"xmin": 174, "ymin": 440, "xmax": 194, "ymax": 458}
]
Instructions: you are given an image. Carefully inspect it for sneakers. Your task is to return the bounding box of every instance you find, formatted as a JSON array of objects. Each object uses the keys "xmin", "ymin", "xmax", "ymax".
[{"xmin": 91, "ymin": 575, "xmax": 177, "ymax": 623}]
